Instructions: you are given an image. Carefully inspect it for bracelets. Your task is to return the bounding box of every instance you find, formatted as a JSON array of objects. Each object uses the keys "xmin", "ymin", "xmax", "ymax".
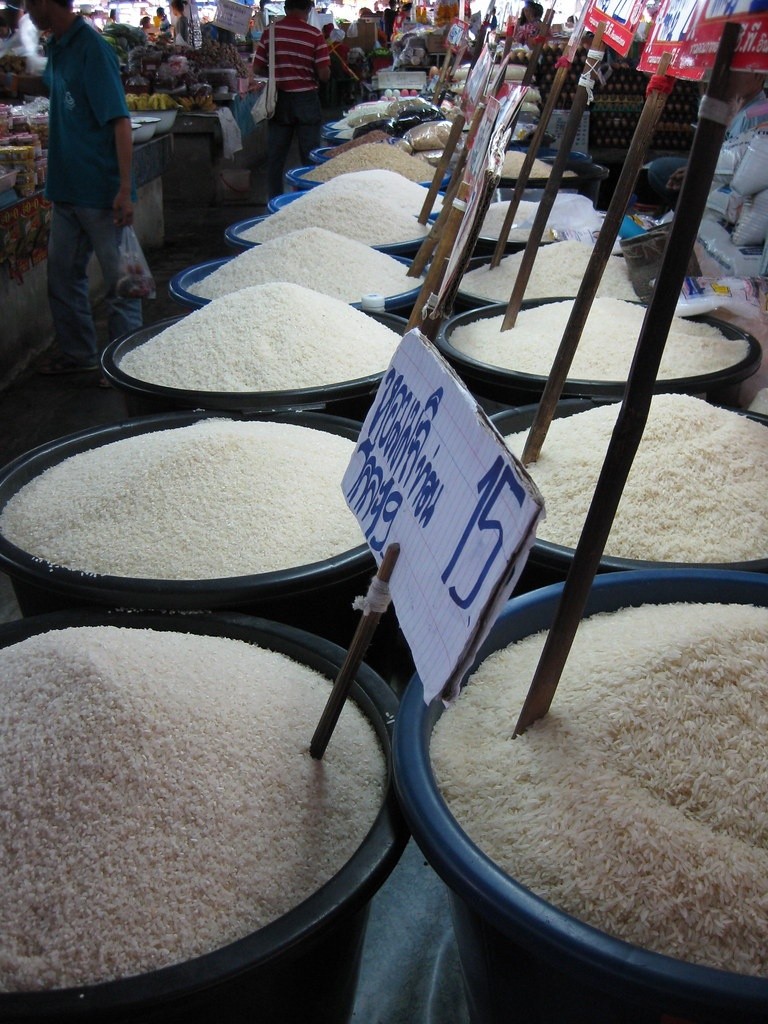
[{"xmin": 6, "ymin": 72, "xmax": 18, "ymax": 96}]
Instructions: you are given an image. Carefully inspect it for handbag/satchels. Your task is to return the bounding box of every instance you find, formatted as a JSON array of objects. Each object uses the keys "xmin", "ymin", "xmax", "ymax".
[
  {"xmin": 115, "ymin": 224, "xmax": 158, "ymax": 301},
  {"xmin": 250, "ymin": 80, "xmax": 278, "ymax": 124}
]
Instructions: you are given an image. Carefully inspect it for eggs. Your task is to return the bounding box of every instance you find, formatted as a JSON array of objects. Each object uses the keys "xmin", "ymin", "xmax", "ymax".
[
  {"xmin": 389, "ymin": 96, "xmax": 396, "ymax": 101},
  {"xmin": 381, "ymin": 96, "xmax": 387, "ymax": 101},
  {"xmin": 385, "ymin": 89, "xmax": 392, "ymax": 97},
  {"xmin": 393, "ymin": 90, "xmax": 400, "ymax": 97},
  {"xmin": 410, "ymin": 90, "xmax": 417, "ymax": 96},
  {"xmin": 430, "ymin": 66, "xmax": 438, "ymax": 77},
  {"xmin": 499, "ymin": 43, "xmax": 695, "ymax": 146},
  {"xmin": 401, "ymin": 90, "xmax": 409, "ymax": 96}
]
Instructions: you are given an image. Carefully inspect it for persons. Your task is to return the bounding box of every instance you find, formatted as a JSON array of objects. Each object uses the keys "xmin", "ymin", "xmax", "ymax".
[
  {"xmin": 384, "ymin": 0, "xmax": 400, "ymax": 41},
  {"xmin": 141, "ymin": 17, "xmax": 150, "ymax": 34},
  {"xmin": 156, "ymin": 7, "xmax": 170, "ymax": 31},
  {"xmin": 666, "ymin": 73, "xmax": 768, "ymax": 189},
  {"xmin": 170, "ymin": 0, "xmax": 188, "ymax": 45},
  {"xmin": 0, "ymin": 0, "xmax": 145, "ymax": 387},
  {"xmin": 253, "ymin": 1, "xmax": 329, "ymax": 199},
  {"xmin": 517, "ymin": 4, "xmax": 550, "ymax": 47},
  {"xmin": 323, "ymin": 25, "xmax": 354, "ymax": 110}
]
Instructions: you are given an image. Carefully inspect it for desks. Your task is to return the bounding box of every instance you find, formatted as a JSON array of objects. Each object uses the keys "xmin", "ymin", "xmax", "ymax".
[
  {"xmin": 0, "ymin": 128, "xmax": 175, "ymax": 404},
  {"xmin": 162, "ymin": 114, "xmax": 225, "ymax": 205}
]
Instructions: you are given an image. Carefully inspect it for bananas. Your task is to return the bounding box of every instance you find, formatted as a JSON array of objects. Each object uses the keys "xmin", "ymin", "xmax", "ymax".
[{"xmin": 126, "ymin": 94, "xmax": 213, "ymax": 110}]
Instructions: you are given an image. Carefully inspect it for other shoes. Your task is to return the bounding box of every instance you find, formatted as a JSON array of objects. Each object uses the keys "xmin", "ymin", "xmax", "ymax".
[
  {"xmin": 37, "ymin": 356, "xmax": 101, "ymax": 374},
  {"xmin": 99, "ymin": 378, "xmax": 113, "ymax": 388}
]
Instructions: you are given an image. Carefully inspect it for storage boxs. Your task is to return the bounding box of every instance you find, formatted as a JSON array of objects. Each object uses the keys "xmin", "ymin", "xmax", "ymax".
[
  {"xmin": 339, "ymin": 22, "xmax": 377, "ymax": 52},
  {"xmin": 428, "ymin": 34, "xmax": 446, "ymax": 52}
]
